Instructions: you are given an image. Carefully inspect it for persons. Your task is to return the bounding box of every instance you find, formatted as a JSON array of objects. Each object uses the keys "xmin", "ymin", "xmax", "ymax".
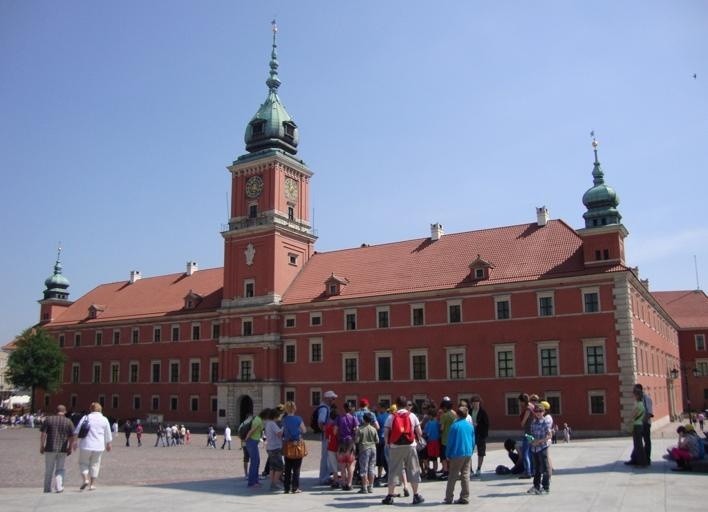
[
  {"xmin": 239, "ymin": 401, "xmax": 307, "ymax": 492},
  {"xmin": 562, "ymin": 422, "xmax": 572, "ymax": 443},
  {"xmin": 154, "ymin": 421, "xmax": 190, "ymax": 448},
  {"xmin": 220, "ymin": 424, "xmax": 231, "ymax": 451},
  {"xmin": 40, "ymin": 405, "xmax": 75, "ymax": 493},
  {"xmin": 311, "ymin": 390, "xmax": 389, "ymax": 504},
  {"xmin": 113, "ymin": 418, "xmax": 120, "ymax": 438},
  {"xmin": 0, "ymin": 408, "xmax": 44, "ymax": 428},
  {"xmin": 383, "ymin": 396, "xmax": 424, "ymax": 505},
  {"xmin": 136, "ymin": 419, "xmax": 142, "ymax": 446},
  {"xmin": 206, "ymin": 424, "xmax": 217, "ymax": 448},
  {"xmin": 631, "ymin": 391, "xmax": 646, "ymax": 467},
  {"xmin": 664, "ymin": 411, "xmax": 708, "ymax": 471},
  {"xmin": 76, "ymin": 402, "xmax": 112, "ymax": 490},
  {"xmin": 407, "ymin": 395, "xmax": 490, "ymax": 504},
  {"xmin": 124, "ymin": 419, "xmax": 132, "ymax": 446},
  {"xmin": 635, "ymin": 384, "xmax": 653, "ymax": 466},
  {"xmin": 384, "ymin": 404, "xmax": 410, "ymax": 497},
  {"xmin": 504, "ymin": 394, "xmax": 557, "ymax": 495}
]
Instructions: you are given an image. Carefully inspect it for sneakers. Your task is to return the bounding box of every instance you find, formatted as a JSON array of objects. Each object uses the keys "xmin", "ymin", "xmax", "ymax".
[
  {"xmin": 437, "ymin": 470, "xmax": 443, "ymax": 473},
  {"xmin": 80, "ymin": 480, "xmax": 89, "ymax": 490},
  {"xmin": 357, "ymin": 488, "xmax": 367, "ymax": 494},
  {"xmin": 670, "ymin": 466, "xmax": 686, "ymax": 473},
  {"xmin": 526, "ymin": 487, "xmax": 538, "ymax": 493},
  {"xmin": 412, "ymin": 495, "xmax": 424, "ymax": 506},
  {"xmin": 270, "ymin": 484, "xmax": 283, "ymax": 491},
  {"xmin": 248, "ymin": 483, "xmax": 264, "ymax": 490},
  {"xmin": 293, "ymin": 489, "xmax": 302, "ymax": 493},
  {"xmin": 439, "ymin": 474, "xmax": 449, "ymax": 481},
  {"xmin": 404, "ymin": 488, "xmax": 408, "ymax": 497},
  {"xmin": 540, "ymin": 489, "xmax": 551, "ymax": 494},
  {"xmin": 518, "ymin": 473, "xmax": 531, "ymax": 480},
  {"xmin": 346, "ymin": 485, "xmax": 352, "ymax": 491},
  {"xmin": 331, "ymin": 481, "xmax": 344, "ymax": 490},
  {"xmin": 421, "ymin": 474, "xmax": 428, "ymax": 482},
  {"xmin": 453, "ymin": 499, "xmax": 468, "ymax": 504},
  {"xmin": 470, "ymin": 469, "xmax": 474, "ymax": 478},
  {"xmin": 367, "ymin": 486, "xmax": 373, "ymax": 494},
  {"xmin": 476, "ymin": 468, "xmax": 480, "ymax": 476},
  {"xmin": 90, "ymin": 485, "xmax": 96, "ymax": 490},
  {"xmin": 341, "ymin": 483, "xmax": 345, "ymax": 490},
  {"xmin": 624, "ymin": 460, "xmax": 638, "ymax": 467},
  {"xmin": 381, "ymin": 495, "xmax": 393, "ymax": 506}
]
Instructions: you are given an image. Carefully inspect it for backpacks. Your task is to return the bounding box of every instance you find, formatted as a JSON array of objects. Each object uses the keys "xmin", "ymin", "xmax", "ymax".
[{"xmin": 389, "ymin": 410, "xmax": 413, "ymax": 445}]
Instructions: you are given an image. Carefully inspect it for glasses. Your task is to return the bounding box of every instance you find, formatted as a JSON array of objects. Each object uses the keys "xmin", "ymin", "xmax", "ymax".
[{"xmin": 533, "ymin": 410, "xmax": 543, "ymax": 413}]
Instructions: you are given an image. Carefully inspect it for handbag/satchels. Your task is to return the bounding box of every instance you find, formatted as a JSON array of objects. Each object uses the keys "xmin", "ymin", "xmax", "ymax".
[
  {"xmin": 281, "ymin": 441, "xmax": 309, "ymax": 460},
  {"xmin": 78, "ymin": 415, "xmax": 91, "ymax": 439}
]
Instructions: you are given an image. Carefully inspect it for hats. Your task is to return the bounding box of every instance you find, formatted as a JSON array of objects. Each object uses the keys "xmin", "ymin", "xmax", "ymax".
[
  {"xmin": 324, "ymin": 391, "xmax": 338, "ymax": 398},
  {"xmin": 386, "ymin": 405, "xmax": 396, "ymax": 412},
  {"xmin": 539, "ymin": 401, "xmax": 550, "ymax": 410},
  {"xmin": 358, "ymin": 399, "xmax": 368, "ymax": 403}
]
